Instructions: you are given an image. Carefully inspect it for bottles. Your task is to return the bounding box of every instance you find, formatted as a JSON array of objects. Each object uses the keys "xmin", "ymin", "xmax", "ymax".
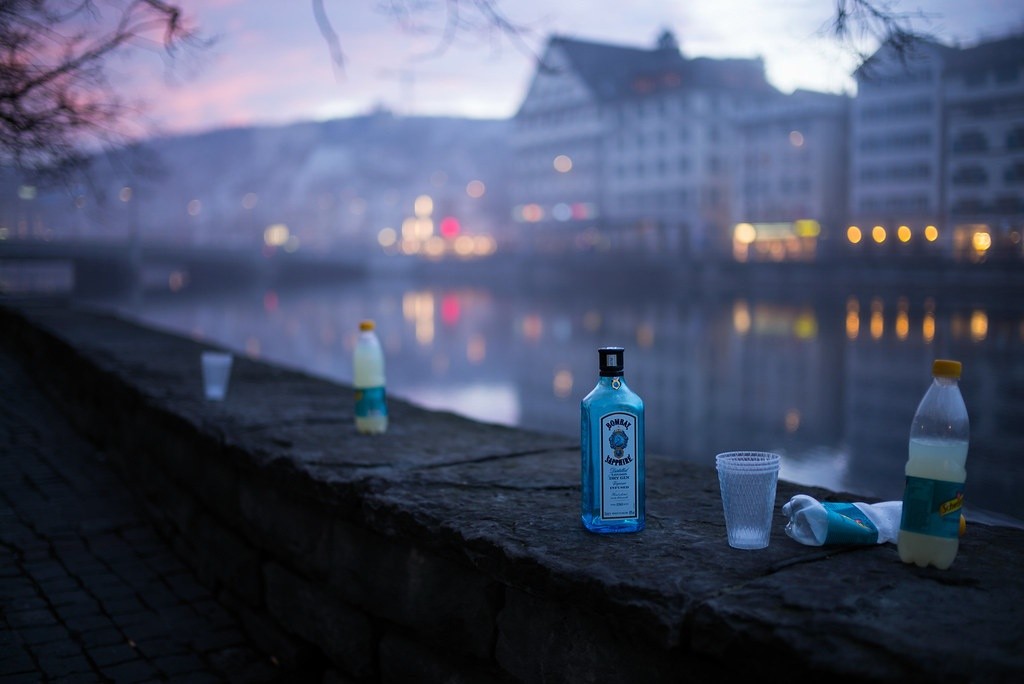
[
  {"xmin": 783, "ymin": 495, "xmax": 903, "ymax": 546},
  {"xmin": 352, "ymin": 320, "xmax": 388, "ymax": 434},
  {"xmin": 898, "ymin": 360, "xmax": 969, "ymax": 571},
  {"xmin": 581, "ymin": 348, "xmax": 646, "ymax": 533}
]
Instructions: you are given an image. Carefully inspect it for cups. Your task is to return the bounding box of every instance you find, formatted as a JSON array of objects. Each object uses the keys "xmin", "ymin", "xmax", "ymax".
[
  {"xmin": 202, "ymin": 353, "xmax": 232, "ymax": 401},
  {"xmin": 716, "ymin": 451, "xmax": 780, "ymax": 549}
]
